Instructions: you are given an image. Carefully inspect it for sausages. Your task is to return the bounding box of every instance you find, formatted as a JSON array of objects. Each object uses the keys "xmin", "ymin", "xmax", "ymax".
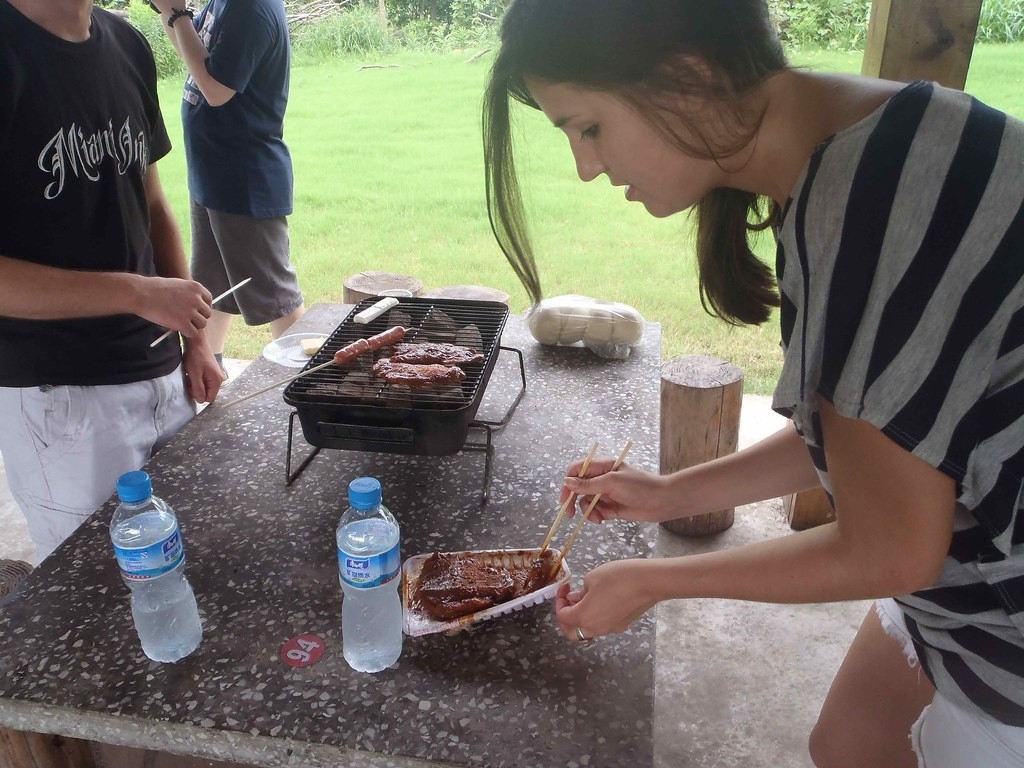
[{"xmin": 334, "ymin": 326, "xmax": 405, "ymax": 364}]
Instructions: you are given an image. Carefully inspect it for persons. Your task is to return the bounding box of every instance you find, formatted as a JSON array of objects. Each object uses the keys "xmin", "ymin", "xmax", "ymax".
[
  {"xmin": 0, "ymin": 0, "xmax": 225, "ymax": 562},
  {"xmin": 480, "ymin": 2, "xmax": 1024, "ymax": 768},
  {"xmin": 143, "ymin": 0, "xmax": 303, "ymax": 381}
]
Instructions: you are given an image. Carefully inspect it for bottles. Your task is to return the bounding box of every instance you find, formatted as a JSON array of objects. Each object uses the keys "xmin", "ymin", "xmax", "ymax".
[
  {"xmin": 109, "ymin": 471, "xmax": 201, "ymax": 662},
  {"xmin": 335, "ymin": 478, "xmax": 403, "ymax": 672}
]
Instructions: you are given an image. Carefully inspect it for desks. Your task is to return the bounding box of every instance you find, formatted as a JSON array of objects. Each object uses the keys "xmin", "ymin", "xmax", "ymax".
[{"xmin": 0, "ymin": 303, "xmax": 664, "ymax": 768}]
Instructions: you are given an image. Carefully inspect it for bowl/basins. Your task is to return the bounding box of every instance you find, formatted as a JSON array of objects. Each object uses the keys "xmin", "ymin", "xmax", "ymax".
[{"xmin": 401, "ymin": 548, "xmax": 572, "ymax": 637}]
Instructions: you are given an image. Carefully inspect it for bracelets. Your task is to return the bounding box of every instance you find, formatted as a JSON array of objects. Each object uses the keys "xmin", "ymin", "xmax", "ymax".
[{"xmin": 168, "ymin": 8, "xmax": 193, "ymax": 29}]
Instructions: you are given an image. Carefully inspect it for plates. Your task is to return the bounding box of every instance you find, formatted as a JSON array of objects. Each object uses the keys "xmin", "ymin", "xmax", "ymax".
[{"xmin": 262, "ymin": 333, "xmax": 330, "ymax": 367}]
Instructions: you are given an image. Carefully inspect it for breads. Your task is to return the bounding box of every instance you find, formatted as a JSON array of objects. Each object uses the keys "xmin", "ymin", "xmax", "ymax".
[{"xmin": 301, "ymin": 338, "xmax": 326, "ymax": 353}]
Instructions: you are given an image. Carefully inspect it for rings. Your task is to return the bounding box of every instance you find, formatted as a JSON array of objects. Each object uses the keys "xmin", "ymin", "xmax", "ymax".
[{"xmin": 575, "ymin": 627, "xmax": 586, "ymax": 641}]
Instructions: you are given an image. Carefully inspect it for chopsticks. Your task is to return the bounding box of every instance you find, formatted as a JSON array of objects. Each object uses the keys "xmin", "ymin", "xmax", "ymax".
[{"xmin": 521, "ymin": 440, "xmax": 633, "ymax": 588}]
